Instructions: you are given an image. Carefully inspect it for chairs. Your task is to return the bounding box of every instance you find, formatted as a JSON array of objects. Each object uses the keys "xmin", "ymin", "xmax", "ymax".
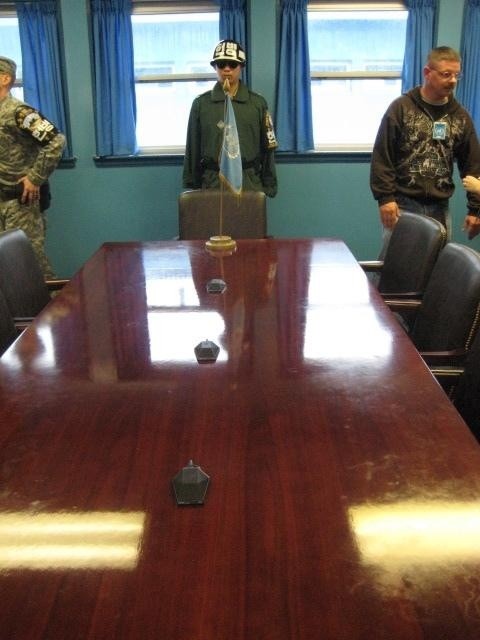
[
  {"xmin": 0, "ymin": 230, "xmax": 73, "ymax": 353},
  {"xmin": 358, "ymin": 210, "xmax": 480, "ymax": 443},
  {"xmin": 178, "ymin": 190, "xmax": 266, "ymax": 238}
]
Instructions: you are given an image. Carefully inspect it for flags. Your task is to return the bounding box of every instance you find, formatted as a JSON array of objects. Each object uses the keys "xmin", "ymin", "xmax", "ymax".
[{"xmin": 218, "ymin": 93, "xmax": 243, "ymax": 197}]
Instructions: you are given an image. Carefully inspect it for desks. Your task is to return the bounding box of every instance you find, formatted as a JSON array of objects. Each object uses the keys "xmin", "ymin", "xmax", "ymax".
[{"xmin": 0, "ymin": 239, "xmax": 480, "ymax": 640}]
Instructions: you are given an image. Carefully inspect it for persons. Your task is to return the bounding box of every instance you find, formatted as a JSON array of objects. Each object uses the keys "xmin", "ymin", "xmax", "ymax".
[
  {"xmin": 0, "ymin": 55, "xmax": 67, "ymax": 301},
  {"xmin": 369, "ymin": 46, "xmax": 480, "ymax": 291},
  {"xmin": 179, "ymin": 39, "xmax": 279, "ymax": 199},
  {"xmin": 462, "ymin": 175, "xmax": 480, "ymax": 195}
]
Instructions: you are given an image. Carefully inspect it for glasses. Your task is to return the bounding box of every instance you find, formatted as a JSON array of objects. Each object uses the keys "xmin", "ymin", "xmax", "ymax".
[{"xmin": 429, "ymin": 67, "xmax": 463, "ymax": 80}]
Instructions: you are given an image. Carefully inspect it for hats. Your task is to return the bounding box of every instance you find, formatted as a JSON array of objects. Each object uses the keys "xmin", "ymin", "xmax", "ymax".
[
  {"xmin": 210, "ymin": 39, "xmax": 247, "ymax": 67},
  {"xmin": 0, "ymin": 55, "xmax": 17, "ymax": 80}
]
